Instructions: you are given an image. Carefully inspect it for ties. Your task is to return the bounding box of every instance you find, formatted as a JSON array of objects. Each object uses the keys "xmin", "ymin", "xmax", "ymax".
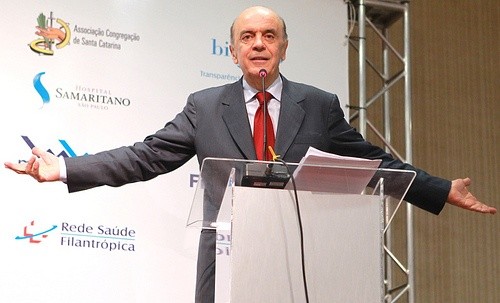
[{"xmin": 252, "ymin": 91, "xmax": 276, "ymax": 161}]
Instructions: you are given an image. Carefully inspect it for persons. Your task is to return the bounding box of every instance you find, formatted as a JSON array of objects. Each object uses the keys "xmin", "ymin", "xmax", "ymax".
[{"xmin": 3, "ymin": 5, "xmax": 497, "ymax": 303}]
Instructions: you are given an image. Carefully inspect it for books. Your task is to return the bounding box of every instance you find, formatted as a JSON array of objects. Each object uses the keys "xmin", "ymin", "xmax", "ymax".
[{"xmin": 285, "ymin": 147, "xmax": 382, "ymax": 194}]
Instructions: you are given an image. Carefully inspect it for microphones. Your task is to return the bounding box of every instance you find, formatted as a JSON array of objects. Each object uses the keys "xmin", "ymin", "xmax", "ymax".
[{"xmin": 259, "ymin": 69, "xmax": 267, "ymax": 161}]
